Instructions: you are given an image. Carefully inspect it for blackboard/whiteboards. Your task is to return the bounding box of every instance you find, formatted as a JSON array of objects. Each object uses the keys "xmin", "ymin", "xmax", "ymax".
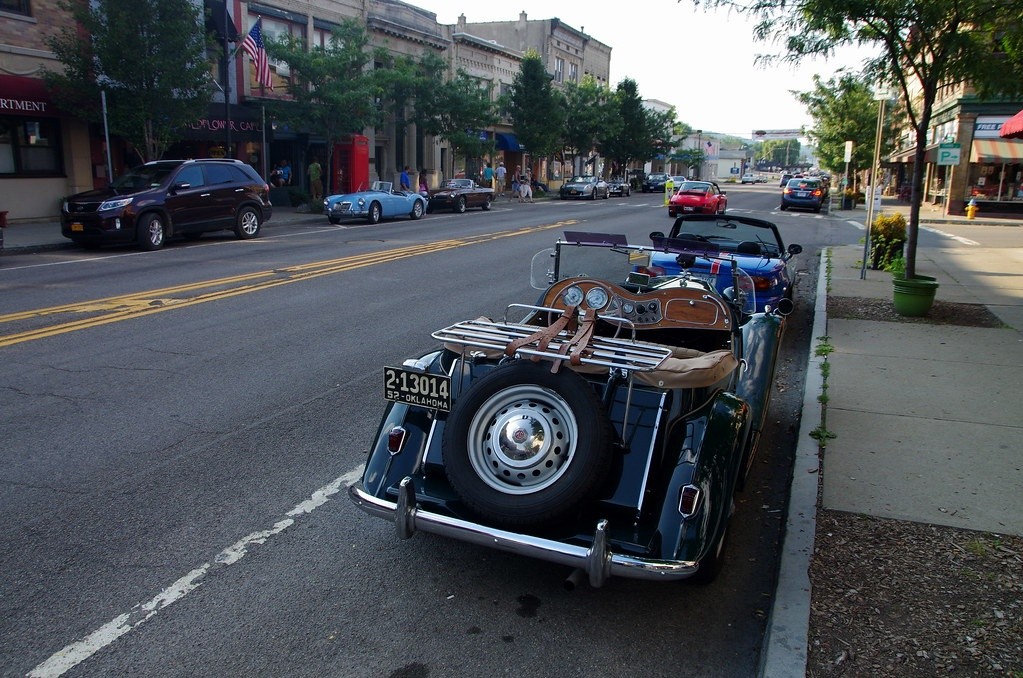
[{"xmin": 865, "ymin": 186, "xmax": 881, "ymax": 211}]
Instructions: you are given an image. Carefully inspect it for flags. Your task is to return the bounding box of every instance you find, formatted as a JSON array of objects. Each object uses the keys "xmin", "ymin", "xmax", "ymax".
[{"xmin": 241, "ymin": 18, "xmax": 274, "ymax": 92}]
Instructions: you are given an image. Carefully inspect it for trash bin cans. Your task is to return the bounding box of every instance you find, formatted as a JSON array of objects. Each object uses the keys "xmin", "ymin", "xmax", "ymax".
[{"xmin": 630, "ymin": 178, "xmax": 637, "ymax": 190}]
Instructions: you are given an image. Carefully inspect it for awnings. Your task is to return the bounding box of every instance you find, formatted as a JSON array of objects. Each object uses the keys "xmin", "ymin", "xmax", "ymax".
[
  {"xmin": 110, "ymin": 94, "xmax": 268, "ymax": 144},
  {"xmin": 0, "ymin": 74, "xmax": 106, "ymax": 123},
  {"xmin": 876, "ymin": 142, "xmax": 937, "ymax": 168},
  {"xmin": 495, "ymin": 132, "xmax": 527, "ymax": 153},
  {"xmin": 999, "ymin": 110, "xmax": 1023, "ymax": 139},
  {"xmin": 969, "ymin": 138, "xmax": 1023, "ymax": 165}
]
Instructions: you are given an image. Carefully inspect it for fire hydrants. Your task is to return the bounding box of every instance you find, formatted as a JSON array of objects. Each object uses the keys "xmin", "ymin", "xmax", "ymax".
[{"xmin": 965, "ymin": 198, "xmax": 979, "ymax": 219}]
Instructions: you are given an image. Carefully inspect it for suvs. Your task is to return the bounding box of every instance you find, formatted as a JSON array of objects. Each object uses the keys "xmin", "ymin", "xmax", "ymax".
[{"xmin": 60, "ymin": 158, "xmax": 274, "ymax": 252}]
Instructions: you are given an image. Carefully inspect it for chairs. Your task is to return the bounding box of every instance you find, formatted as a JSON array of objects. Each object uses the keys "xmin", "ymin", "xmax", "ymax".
[
  {"xmin": 736, "ymin": 241, "xmax": 760, "ymax": 255},
  {"xmin": 678, "ymin": 232, "xmax": 698, "ymax": 241}
]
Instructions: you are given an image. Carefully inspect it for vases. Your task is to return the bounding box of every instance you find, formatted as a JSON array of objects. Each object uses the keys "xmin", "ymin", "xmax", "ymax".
[{"xmin": 891, "ymin": 270, "xmax": 939, "ymax": 316}]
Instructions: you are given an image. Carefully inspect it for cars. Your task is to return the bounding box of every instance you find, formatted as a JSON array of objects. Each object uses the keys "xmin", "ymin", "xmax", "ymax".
[
  {"xmin": 323, "ymin": 181, "xmax": 428, "ymax": 225},
  {"xmin": 630, "ymin": 214, "xmax": 803, "ymax": 313},
  {"xmin": 780, "ymin": 169, "xmax": 832, "ymax": 214},
  {"xmin": 350, "ymin": 231, "xmax": 792, "ymax": 591},
  {"xmin": 422, "ymin": 179, "xmax": 497, "ymax": 214},
  {"xmin": 672, "ymin": 176, "xmax": 689, "ymax": 191},
  {"xmin": 741, "ymin": 172, "xmax": 755, "ymax": 185},
  {"xmin": 642, "ymin": 172, "xmax": 674, "ymax": 194},
  {"xmin": 606, "ymin": 178, "xmax": 632, "ymax": 198},
  {"xmin": 668, "ymin": 181, "xmax": 729, "ymax": 216},
  {"xmin": 560, "ymin": 176, "xmax": 610, "ymax": 200}
]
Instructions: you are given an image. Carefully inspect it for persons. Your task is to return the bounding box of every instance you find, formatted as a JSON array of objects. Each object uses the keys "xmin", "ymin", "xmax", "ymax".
[
  {"xmin": 307, "ymin": 156, "xmax": 323, "ymax": 199},
  {"xmin": 399, "ymin": 166, "xmax": 410, "ymax": 191},
  {"xmin": 494, "ymin": 162, "xmax": 507, "ymax": 197},
  {"xmin": 270, "ymin": 159, "xmax": 292, "ymax": 188},
  {"xmin": 509, "ymin": 165, "xmax": 535, "ymax": 203},
  {"xmin": 482, "ymin": 163, "xmax": 496, "ymax": 188},
  {"xmin": 531, "ymin": 174, "xmax": 548, "ymax": 192},
  {"xmin": 419, "ymin": 169, "xmax": 432, "ymax": 217}
]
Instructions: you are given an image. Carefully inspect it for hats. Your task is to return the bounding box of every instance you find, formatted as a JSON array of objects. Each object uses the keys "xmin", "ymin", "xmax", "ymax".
[
  {"xmin": 517, "ymin": 165, "xmax": 522, "ymax": 168},
  {"xmin": 527, "ymin": 167, "xmax": 531, "ymax": 171},
  {"xmin": 500, "ymin": 161, "xmax": 504, "ymax": 165}
]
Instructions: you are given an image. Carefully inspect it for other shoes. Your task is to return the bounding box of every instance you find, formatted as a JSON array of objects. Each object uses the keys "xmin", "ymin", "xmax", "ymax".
[
  {"xmin": 509, "ymin": 199, "xmax": 536, "ymax": 203},
  {"xmin": 497, "ymin": 193, "xmax": 500, "ymax": 197},
  {"xmin": 502, "ymin": 193, "xmax": 505, "ymax": 197}
]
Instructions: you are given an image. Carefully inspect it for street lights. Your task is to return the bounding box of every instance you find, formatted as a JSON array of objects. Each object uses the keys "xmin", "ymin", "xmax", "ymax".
[{"xmin": 697, "ymin": 129, "xmax": 703, "ymax": 180}]
